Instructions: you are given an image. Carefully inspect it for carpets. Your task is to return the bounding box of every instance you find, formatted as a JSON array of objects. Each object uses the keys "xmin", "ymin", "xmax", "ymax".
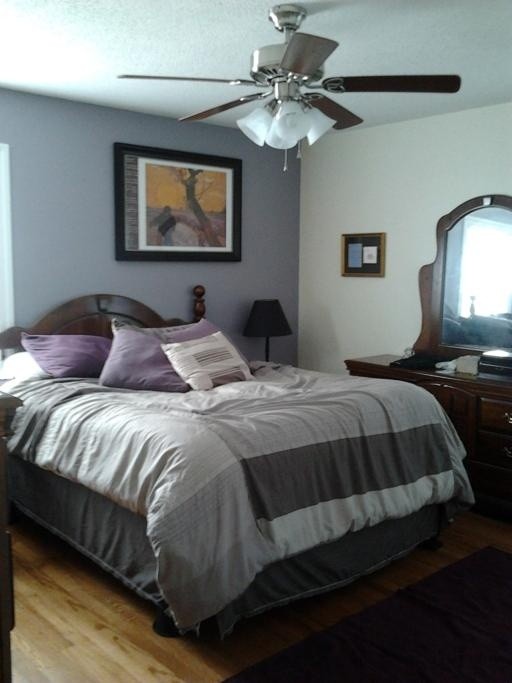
[{"xmin": 221, "ymin": 546, "xmax": 511, "ymax": 682}]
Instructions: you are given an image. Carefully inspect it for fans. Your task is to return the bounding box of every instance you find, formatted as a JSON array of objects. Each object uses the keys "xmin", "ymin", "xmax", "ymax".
[{"xmin": 117, "ymin": 3, "xmax": 461, "ymax": 130}]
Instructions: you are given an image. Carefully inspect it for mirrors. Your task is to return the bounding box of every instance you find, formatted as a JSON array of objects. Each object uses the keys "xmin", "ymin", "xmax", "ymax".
[{"xmin": 407, "ymin": 194, "xmax": 512, "ymax": 369}]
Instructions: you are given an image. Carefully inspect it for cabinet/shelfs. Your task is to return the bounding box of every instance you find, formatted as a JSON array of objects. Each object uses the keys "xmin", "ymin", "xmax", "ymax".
[{"xmin": 342, "ymin": 354, "xmax": 512, "ymax": 520}]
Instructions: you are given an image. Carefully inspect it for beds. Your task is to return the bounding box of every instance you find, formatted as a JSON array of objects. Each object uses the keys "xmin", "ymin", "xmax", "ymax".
[{"xmin": 0, "ymin": 281, "xmax": 453, "ymax": 637}]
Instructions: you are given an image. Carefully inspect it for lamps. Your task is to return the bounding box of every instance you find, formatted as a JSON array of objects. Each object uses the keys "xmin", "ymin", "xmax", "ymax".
[
  {"xmin": 237, "ymin": 80, "xmax": 337, "ymax": 169},
  {"xmin": 243, "ymin": 297, "xmax": 292, "ymax": 362}
]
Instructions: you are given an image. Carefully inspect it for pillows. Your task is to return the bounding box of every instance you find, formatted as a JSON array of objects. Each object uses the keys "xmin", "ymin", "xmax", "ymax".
[{"xmin": 0, "ymin": 315, "xmax": 258, "ymax": 401}]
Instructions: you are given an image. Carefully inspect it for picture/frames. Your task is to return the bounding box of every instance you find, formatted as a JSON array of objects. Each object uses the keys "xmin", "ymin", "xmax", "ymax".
[
  {"xmin": 339, "ymin": 233, "xmax": 385, "ymax": 278},
  {"xmin": 111, "ymin": 139, "xmax": 243, "ymax": 263}
]
